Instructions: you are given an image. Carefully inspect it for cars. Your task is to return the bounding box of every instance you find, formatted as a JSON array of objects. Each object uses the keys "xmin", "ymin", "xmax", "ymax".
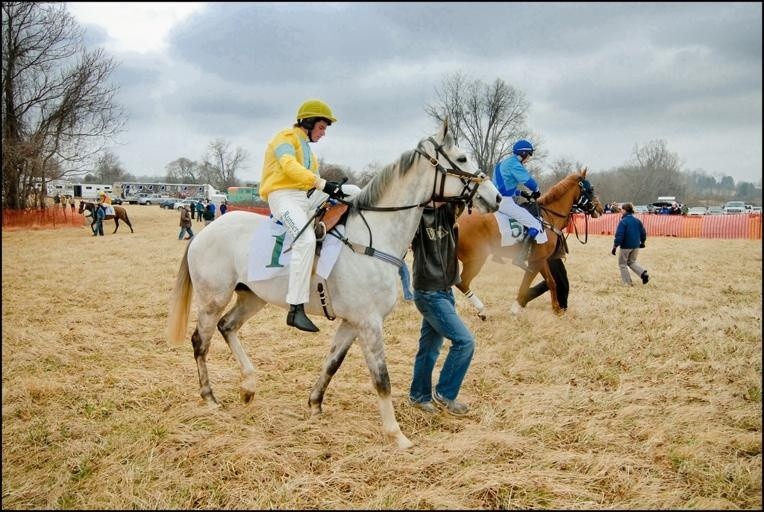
[
  {"xmin": 24, "ymin": 181, "xmax": 221, "ymax": 213},
  {"xmin": 614, "ymin": 195, "xmax": 763, "ymax": 216}
]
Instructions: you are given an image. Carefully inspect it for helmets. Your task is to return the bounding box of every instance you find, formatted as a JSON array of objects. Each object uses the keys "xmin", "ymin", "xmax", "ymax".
[
  {"xmin": 296, "ymin": 100, "xmax": 336, "ymax": 125},
  {"xmin": 513, "ymin": 140, "xmax": 533, "ymax": 154}
]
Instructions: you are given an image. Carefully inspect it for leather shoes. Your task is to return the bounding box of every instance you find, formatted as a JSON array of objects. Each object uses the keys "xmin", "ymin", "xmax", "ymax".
[{"xmin": 287, "ymin": 304, "xmax": 319, "ymax": 332}]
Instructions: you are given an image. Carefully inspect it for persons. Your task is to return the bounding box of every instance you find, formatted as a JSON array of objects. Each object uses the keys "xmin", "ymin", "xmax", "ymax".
[
  {"xmin": 601, "ymin": 199, "xmax": 688, "ymax": 237},
  {"xmin": 189, "ymin": 198, "xmax": 227, "ymax": 223},
  {"xmin": 53, "ymin": 193, "xmax": 60, "ymax": 212},
  {"xmin": 256, "ymin": 98, "xmax": 344, "ymax": 332},
  {"xmin": 91, "ymin": 204, "xmax": 104, "ymax": 236},
  {"xmin": 407, "ymin": 199, "xmax": 474, "ymax": 419},
  {"xmin": 492, "ymin": 140, "xmax": 542, "ymax": 274},
  {"xmin": 610, "ymin": 201, "xmax": 649, "ymax": 286},
  {"xmin": 525, "ymin": 234, "xmax": 570, "ymax": 312},
  {"xmin": 98, "ymin": 191, "xmax": 111, "ymax": 205},
  {"xmin": 60, "ymin": 195, "xmax": 67, "ymax": 220},
  {"xmin": 68, "ymin": 195, "xmax": 75, "ymax": 213},
  {"xmin": 178, "ymin": 204, "xmax": 193, "ymax": 240}
]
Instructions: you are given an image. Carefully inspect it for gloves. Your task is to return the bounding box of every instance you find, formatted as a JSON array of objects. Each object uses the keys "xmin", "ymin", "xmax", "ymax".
[
  {"xmin": 533, "ymin": 192, "xmax": 540, "ymax": 198},
  {"xmin": 613, "ymin": 248, "xmax": 615, "ymax": 255},
  {"xmin": 323, "ymin": 181, "xmax": 343, "ymax": 197}
]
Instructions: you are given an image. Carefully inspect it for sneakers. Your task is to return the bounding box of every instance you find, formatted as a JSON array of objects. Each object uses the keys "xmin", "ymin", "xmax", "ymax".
[
  {"xmin": 409, "ymin": 391, "xmax": 468, "ymax": 415},
  {"xmin": 643, "ymin": 275, "xmax": 648, "ymax": 283}
]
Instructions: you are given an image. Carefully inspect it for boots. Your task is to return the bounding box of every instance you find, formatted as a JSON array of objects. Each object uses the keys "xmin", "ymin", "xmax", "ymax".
[{"xmin": 513, "ymin": 234, "xmax": 533, "ymax": 273}]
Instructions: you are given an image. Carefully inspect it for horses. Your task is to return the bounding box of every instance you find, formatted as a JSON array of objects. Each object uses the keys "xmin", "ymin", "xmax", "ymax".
[
  {"xmin": 164, "ymin": 114, "xmax": 503, "ymax": 455},
  {"xmin": 78, "ymin": 200, "xmax": 133, "ymax": 233},
  {"xmin": 453, "ymin": 165, "xmax": 605, "ymax": 322}
]
